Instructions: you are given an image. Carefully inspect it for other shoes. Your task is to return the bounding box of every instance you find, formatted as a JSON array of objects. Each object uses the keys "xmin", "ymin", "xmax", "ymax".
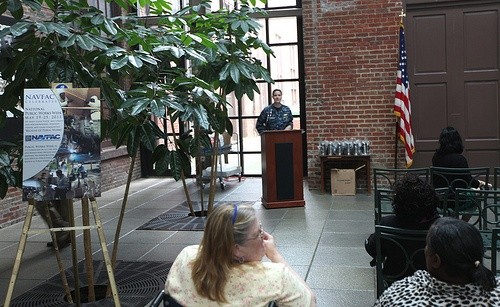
[{"xmin": 47, "ymin": 240, "xmax": 71, "ymax": 247}]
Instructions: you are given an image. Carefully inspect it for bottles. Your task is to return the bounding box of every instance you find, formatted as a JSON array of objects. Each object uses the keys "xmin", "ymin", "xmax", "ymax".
[{"xmin": 320, "ymin": 141, "xmax": 370, "ymax": 155}]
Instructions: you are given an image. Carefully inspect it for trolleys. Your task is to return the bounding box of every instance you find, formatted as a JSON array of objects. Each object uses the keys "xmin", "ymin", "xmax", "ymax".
[{"xmin": 200, "ymin": 132, "xmax": 242, "ymax": 190}]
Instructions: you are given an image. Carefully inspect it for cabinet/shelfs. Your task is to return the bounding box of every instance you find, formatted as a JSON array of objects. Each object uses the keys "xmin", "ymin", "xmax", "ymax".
[{"xmin": 199, "ymin": 133, "xmax": 242, "ymax": 189}]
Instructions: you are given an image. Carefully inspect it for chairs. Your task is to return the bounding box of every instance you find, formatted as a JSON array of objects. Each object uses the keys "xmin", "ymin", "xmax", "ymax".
[{"xmin": 373, "ymin": 168, "xmax": 500, "ymax": 300}]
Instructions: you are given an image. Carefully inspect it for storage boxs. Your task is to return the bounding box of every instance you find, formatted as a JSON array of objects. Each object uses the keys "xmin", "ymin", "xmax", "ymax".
[{"xmin": 330, "ymin": 165, "xmax": 366, "ymax": 196}]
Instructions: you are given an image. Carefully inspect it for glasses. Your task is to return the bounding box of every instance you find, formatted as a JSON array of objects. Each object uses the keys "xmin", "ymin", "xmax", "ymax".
[{"xmin": 236, "ymin": 219, "xmax": 263, "ymax": 240}]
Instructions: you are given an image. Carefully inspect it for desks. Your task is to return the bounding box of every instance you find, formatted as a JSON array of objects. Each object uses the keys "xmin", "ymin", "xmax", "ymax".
[{"xmin": 320, "ymin": 155, "xmax": 371, "ymax": 194}]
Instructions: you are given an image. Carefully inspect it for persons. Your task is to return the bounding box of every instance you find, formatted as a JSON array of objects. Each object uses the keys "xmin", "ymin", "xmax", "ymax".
[
  {"xmin": 365, "ymin": 171, "xmax": 446, "ymax": 287},
  {"xmin": 255, "ymin": 89, "xmax": 294, "ymax": 135},
  {"xmin": 369, "ymin": 216, "xmax": 500, "ymax": 307},
  {"xmin": 432, "ymin": 125, "xmax": 493, "ymax": 224},
  {"xmin": 163, "ymin": 201, "xmax": 318, "ymax": 307}
]
